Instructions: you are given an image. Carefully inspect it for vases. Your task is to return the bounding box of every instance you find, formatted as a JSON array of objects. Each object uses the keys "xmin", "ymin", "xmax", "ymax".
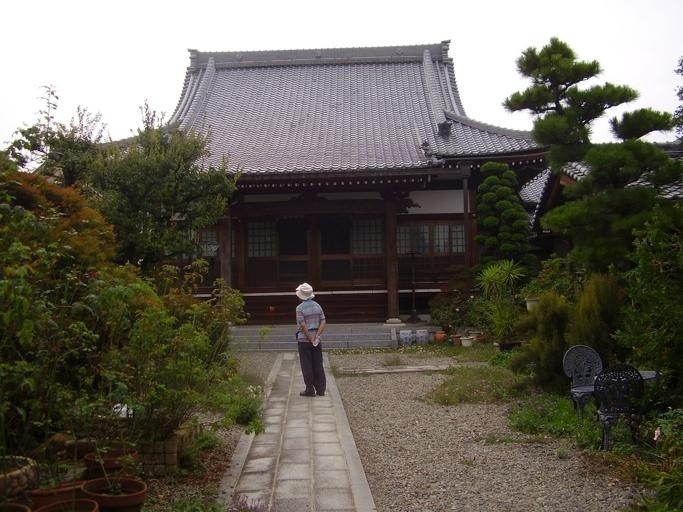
[{"xmin": 400, "ymin": 329, "xmax": 427, "ymax": 346}]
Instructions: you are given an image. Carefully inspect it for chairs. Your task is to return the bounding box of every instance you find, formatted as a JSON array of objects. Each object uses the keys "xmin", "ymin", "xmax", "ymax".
[{"xmin": 562, "ymin": 344, "xmax": 644, "ymax": 454}]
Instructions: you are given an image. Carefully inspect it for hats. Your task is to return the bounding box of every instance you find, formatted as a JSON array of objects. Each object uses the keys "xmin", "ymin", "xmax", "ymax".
[{"xmin": 296, "ymin": 283, "xmax": 315, "ymax": 301}]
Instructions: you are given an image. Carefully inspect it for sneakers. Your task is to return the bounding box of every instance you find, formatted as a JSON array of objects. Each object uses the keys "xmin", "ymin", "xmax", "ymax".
[
  {"xmin": 300, "ymin": 391, "xmax": 316, "ymax": 396},
  {"xmin": 316, "ymin": 391, "xmax": 325, "ymax": 396}
]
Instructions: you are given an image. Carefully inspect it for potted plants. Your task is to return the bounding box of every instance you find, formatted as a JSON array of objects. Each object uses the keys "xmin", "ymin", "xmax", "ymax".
[
  {"xmin": 429, "ymin": 259, "xmax": 544, "ymax": 352},
  {"xmin": 3, "ymin": 204, "xmax": 165, "ymax": 512}
]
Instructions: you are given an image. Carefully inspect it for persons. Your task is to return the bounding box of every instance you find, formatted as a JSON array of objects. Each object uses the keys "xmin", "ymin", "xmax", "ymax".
[{"xmin": 294, "ymin": 282, "xmax": 327, "ymax": 397}]
